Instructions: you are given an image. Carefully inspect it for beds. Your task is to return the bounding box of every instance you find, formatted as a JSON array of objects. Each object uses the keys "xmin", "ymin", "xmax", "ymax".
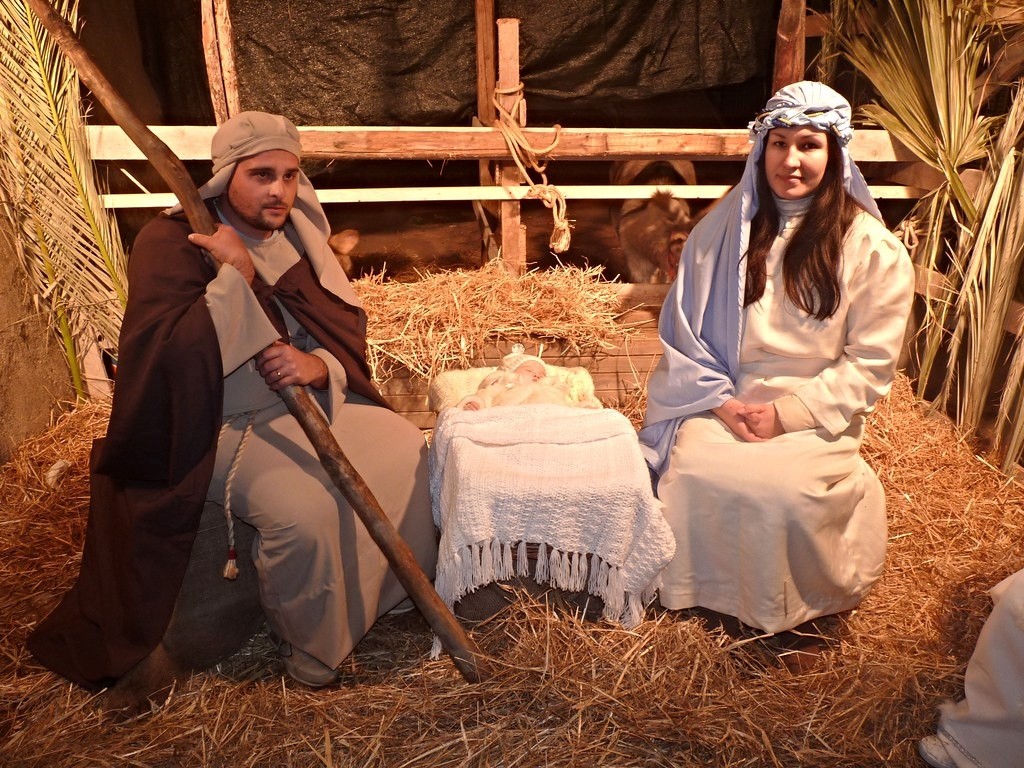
[{"xmin": 429, "ymin": 365, "xmax": 636, "ymax": 621}]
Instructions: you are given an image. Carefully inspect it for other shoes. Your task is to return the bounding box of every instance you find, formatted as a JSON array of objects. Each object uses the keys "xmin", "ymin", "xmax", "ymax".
[
  {"xmin": 920, "ymin": 735, "xmax": 958, "ymax": 768},
  {"xmin": 269, "ymin": 630, "xmax": 340, "ymax": 686},
  {"xmin": 388, "ymin": 597, "xmax": 416, "ymax": 614}
]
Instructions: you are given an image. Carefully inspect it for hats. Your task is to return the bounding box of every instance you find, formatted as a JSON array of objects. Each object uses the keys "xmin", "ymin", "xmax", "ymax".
[
  {"xmin": 498, "ymin": 352, "xmax": 547, "ymax": 374},
  {"xmin": 749, "ymin": 80, "xmax": 853, "ymax": 145},
  {"xmin": 211, "ymin": 111, "xmax": 302, "ymax": 173}
]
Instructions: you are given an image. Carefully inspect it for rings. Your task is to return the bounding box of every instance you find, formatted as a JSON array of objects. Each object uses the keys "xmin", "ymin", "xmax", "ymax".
[{"xmin": 275, "ymin": 370, "xmax": 281, "ymax": 378}]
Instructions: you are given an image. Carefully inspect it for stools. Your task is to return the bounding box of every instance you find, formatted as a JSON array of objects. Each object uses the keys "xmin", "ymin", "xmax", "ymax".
[{"xmin": 88, "ymin": 451, "xmax": 265, "ymax": 675}]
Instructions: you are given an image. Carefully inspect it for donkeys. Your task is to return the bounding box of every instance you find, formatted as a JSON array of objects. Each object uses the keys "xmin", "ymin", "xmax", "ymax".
[{"xmin": 612, "ymin": 160, "xmax": 694, "ymax": 285}]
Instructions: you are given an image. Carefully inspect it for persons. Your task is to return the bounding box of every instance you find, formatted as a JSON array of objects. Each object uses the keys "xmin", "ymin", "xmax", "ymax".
[
  {"xmin": 453, "ymin": 350, "xmax": 550, "ymax": 410},
  {"xmin": 26, "ymin": 109, "xmax": 444, "ymax": 691},
  {"xmin": 640, "ymin": 82, "xmax": 917, "ymax": 632}
]
